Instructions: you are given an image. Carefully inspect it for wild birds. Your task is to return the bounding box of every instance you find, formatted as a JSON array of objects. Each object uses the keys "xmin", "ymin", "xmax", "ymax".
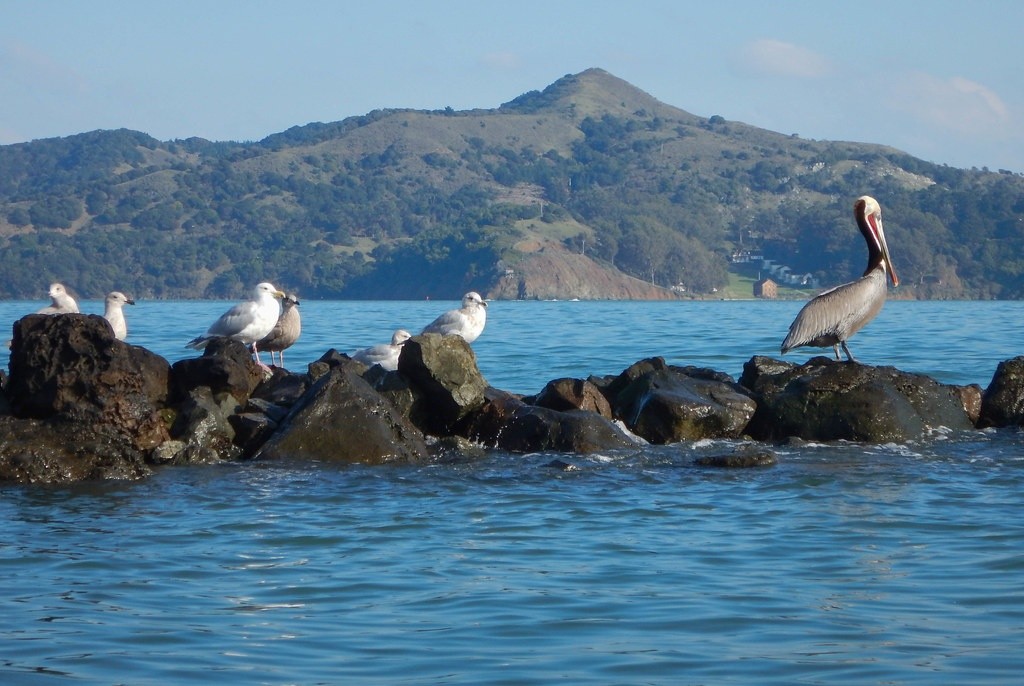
[
  {"xmin": 184, "ymin": 281, "xmax": 302, "ymax": 377},
  {"xmin": 351, "ymin": 292, "xmax": 490, "ymax": 373},
  {"xmin": 780, "ymin": 194, "xmax": 900, "ymax": 364},
  {"xmin": 100, "ymin": 290, "xmax": 137, "ymax": 341},
  {"xmin": 36, "ymin": 282, "xmax": 81, "ymax": 317}
]
